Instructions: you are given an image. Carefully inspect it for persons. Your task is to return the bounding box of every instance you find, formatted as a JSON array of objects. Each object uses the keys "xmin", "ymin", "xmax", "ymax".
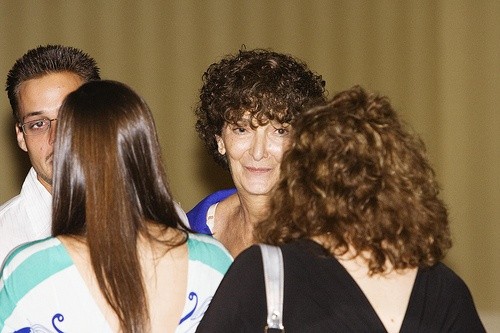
[
  {"xmin": 185, "ymin": 48, "xmax": 328, "ymax": 262},
  {"xmin": 0, "ymin": 80, "xmax": 235, "ymax": 333},
  {"xmin": 195, "ymin": 86, "xmax": 489, "ymax": 333},
  {"xmin": 0, "ymin": 45, "xmax": 193, "ymax": 272}
]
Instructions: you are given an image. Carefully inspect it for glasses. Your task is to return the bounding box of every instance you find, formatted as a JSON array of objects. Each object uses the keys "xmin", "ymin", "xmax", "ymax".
[{"xmin": 19, "ymin": 117, "xmax": 60, "ymax": 136}]
